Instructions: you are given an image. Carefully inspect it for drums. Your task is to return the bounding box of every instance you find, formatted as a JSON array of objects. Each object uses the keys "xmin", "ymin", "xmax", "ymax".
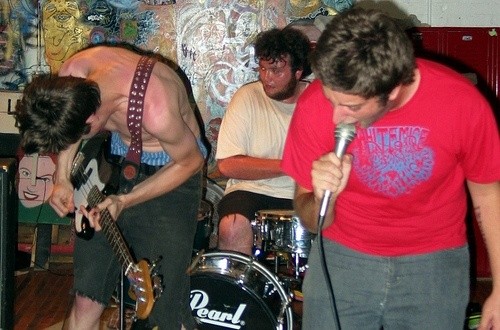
[
  {"xmin": 188, "ymin": 250, "xmax": 292, "ymax": 330},
  {"xmin": 250, "ymin": 212, "xmax": 315, "ymax": 281}
]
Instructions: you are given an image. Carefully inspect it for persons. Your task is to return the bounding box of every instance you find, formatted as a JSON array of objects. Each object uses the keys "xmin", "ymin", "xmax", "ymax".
[
  {"xmin": 14, "ymin": 45, "xmax": 208, "ymax": 330},
  {"xmin": 214, "ymin": 28, "xmax": 312, "ymax": 256},
  {"xmin": 281, "ymin": 8, "xmax": 500, "ymax": 330}
]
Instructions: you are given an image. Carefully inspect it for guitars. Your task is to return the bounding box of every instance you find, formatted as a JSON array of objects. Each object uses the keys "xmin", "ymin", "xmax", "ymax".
[{"xmin": 65, "ymin": 129, "xmax": 163, "ymax": 320}]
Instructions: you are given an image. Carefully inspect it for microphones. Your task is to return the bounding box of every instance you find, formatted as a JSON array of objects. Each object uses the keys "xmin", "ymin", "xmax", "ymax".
[{"xmin": 318, "ymin": 122, "xmax": 355, "ymax": 226}]
